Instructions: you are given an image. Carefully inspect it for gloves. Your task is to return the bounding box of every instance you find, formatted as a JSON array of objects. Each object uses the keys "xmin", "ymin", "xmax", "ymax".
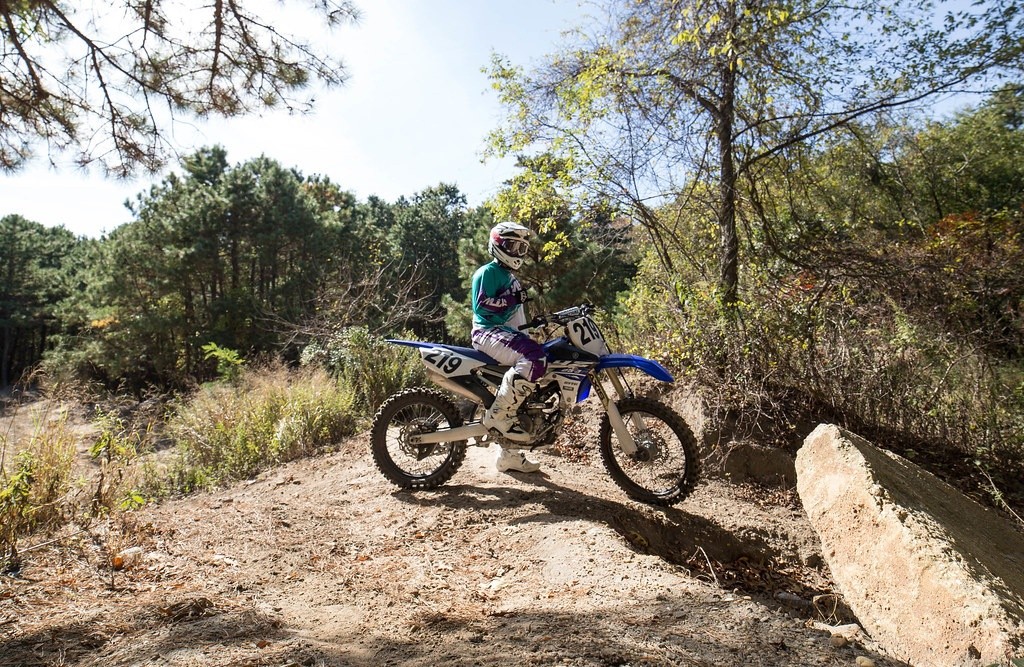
[{"xmin": 502, "ymin": 288, "xmax": 534, "ymax": 307}]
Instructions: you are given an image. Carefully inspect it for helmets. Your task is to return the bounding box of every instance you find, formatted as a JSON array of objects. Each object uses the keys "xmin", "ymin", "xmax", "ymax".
[{"xmin": 488, "ymin": 221, "xmax": 537, "ymax": 271}]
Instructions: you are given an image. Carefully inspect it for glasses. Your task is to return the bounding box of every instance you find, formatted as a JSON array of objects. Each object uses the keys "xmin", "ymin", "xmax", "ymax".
[{"xmin": 499, "ymin": 236, "xmax": 530, "ymax": 256}]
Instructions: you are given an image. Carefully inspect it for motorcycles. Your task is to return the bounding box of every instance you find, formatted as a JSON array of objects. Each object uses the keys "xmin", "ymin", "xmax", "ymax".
[{"xmin": 368, "ymin": 304, "xmax": 702, "ymax": 508}]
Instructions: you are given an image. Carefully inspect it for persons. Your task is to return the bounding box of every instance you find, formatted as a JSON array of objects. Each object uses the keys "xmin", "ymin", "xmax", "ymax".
[{"xmin": 470, "ymin": 221, "xmax": 548, "ymax": 474}]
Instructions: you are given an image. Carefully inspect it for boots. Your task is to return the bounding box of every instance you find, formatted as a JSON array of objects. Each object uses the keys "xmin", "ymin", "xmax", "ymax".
[
  {"xmin": 496, "ymin": 448, "xmax": 541, "ymax": 473},
  {"xmin": 482, "ymin": 366, "xmax": 536, "ymax": 441}
]
